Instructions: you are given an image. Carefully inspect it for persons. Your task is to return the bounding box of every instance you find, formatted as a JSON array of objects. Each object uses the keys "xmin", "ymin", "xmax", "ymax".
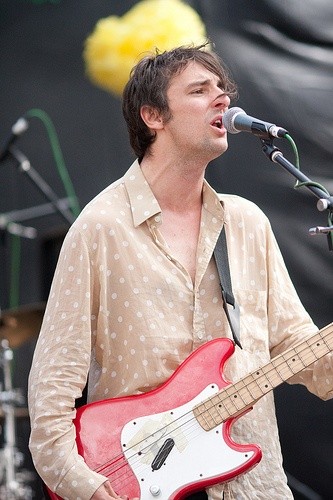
[{"xmin": 27, "ymin": 40, "xmax": 332, "ymax": 500}]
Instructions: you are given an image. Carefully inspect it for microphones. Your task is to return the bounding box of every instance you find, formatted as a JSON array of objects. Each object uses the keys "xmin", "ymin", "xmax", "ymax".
[
  {"xmin": 0, "ymin": 117, "xmax": 28, "ymax": 165},
  {"xmin": 222, "ymin": 106, "xmax": 289, "ymax": 139}
]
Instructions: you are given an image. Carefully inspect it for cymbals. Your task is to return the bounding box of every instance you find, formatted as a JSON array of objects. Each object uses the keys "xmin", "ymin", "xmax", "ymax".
[{"xmin": 0, "ymin": 301, "xmax": 46, "ymax": 348}]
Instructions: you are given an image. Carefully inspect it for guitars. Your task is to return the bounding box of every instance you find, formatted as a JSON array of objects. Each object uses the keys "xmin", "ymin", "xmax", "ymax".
[{"xmin": 43, "ymin": 316, "xmax": 333, "ymax": 500}]
